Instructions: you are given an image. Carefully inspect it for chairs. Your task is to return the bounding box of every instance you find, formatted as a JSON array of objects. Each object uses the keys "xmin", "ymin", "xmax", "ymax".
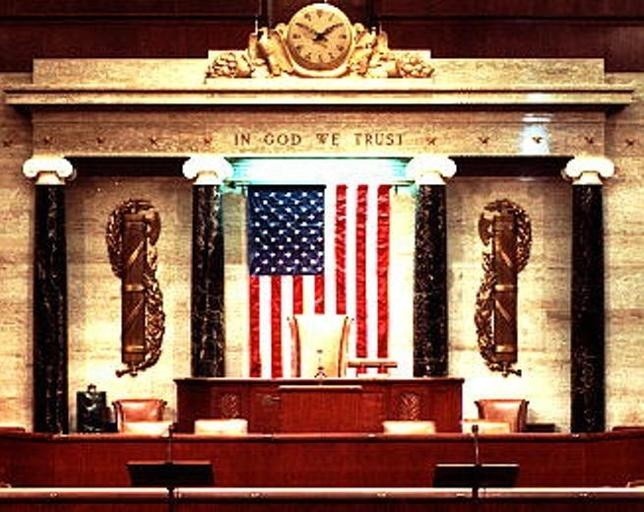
[
  {"xmin": 286, "ymin": 306, "xmax": 361, "ymax": 378},
  {"xmin": 123, "ymin": 457, "xmax": 215, "ymax": 487},
  {"xmin": 190, "ymin": 417, "xmax": 250, "ymax": 437},
  {"xmin": 475, "ymin": 394, "xmax": 533, "ymax": 433},
  {"xmin": 428, "ymin": 460, "xmax": 521, "ymax": 489},
  {"xmin": 455, "ymin": 415, "xmax": 512, "ymax": 437},
  {"xmin": 122, "ymin": 414, "xmax": 175, "ymax": 437},
  {"xmin": 381, "ymin": 417, "xmax": 438, "ymax": 435},
  {"xmin": 111, "ymin": 393, "xmax": 171, "ymax": 436}
]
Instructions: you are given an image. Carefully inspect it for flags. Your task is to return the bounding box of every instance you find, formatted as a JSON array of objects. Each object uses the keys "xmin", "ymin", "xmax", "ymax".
[{"xmin": 246, "ymin": 182, "xmax": 390, "ymax": 377}]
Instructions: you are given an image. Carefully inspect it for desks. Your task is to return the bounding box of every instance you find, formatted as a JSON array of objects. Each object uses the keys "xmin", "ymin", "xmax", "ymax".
[
  {"xmin": 0, "ymin": 425, "xmax": 644, "ymax": 487},
  {"xmin": 171, "ymin": 370, "xmax": 467, "ymax": 433},
  {"xmin": 0, "ymin": 485, "xmax": 644, "ymax": 512}
]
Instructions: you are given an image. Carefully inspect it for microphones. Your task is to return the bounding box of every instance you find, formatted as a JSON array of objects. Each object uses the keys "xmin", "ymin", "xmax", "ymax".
[
  {"xmin": 472, "ymin": 425, "xmax": 480, "ymax": 491},
  {"xmin": 313, "ymin": 349, "xmax": 328, "ymax": 379},
  {"xmin": 168, "ymin": 425, "xmax": 174, "ymax": 493}
]
